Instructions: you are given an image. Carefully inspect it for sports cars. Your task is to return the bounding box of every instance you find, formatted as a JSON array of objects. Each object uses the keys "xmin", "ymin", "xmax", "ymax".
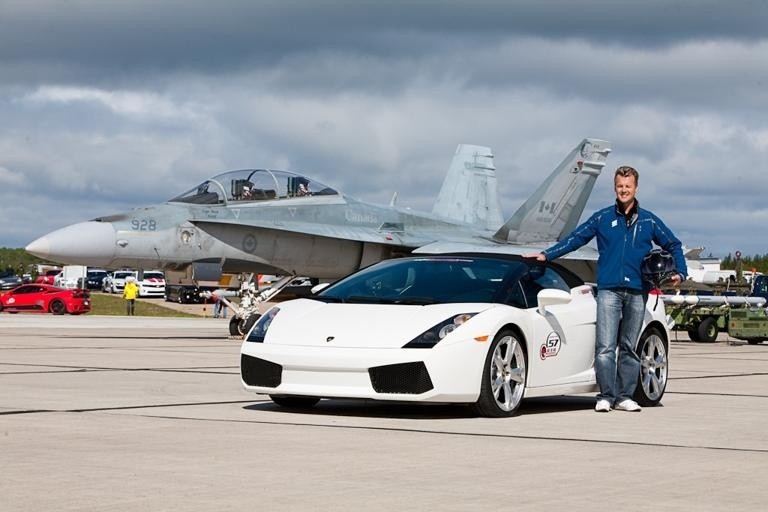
[
  {"xmin": 0, "ymin": 284, "xmax": 91, "ymax": 315},
  {"xmin": 240, "ymin": 253, "xmax": 671, "ymax": 418}
]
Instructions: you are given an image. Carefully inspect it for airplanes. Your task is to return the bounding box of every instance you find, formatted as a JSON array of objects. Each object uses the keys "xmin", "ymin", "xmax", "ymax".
[{"xmin": 24, "ymin": 135, "xmax": 763, "ymax": 306}]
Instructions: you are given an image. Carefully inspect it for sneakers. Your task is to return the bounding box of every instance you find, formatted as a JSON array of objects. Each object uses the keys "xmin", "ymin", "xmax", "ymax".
[
  {"xmin": 594, "ymin": 400, "xmax": 610, "ymax": 412},
  {"xmin": 614, "ymin": 396, "xmax": 641, "ymax": 411}
]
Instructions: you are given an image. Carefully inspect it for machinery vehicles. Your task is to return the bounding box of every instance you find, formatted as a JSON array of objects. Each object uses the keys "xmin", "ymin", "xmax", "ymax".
[{"xmin": 664, "ymin": 275, "xmax": 768, "ymax": 344}]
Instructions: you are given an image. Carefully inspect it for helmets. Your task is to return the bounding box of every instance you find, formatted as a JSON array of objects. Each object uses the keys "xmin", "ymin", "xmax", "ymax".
[{"xmin": 640, "ymin": 248, "xmax": 676, "ymax": 290}]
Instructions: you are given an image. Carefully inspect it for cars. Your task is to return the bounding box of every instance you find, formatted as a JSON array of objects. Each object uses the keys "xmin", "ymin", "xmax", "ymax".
[{"xmin": 0, "ymin": 266, "xmax": 218, "ymax": 303}]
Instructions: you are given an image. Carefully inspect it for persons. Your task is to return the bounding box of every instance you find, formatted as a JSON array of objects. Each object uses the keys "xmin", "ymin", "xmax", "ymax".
[
  {"xmin": 122, "ymin": 276, "xmax": 139, "ymax": 316},
  {"xmin": 520, "ymin": 166, "xmax": 688, "ymax": 413}
]
